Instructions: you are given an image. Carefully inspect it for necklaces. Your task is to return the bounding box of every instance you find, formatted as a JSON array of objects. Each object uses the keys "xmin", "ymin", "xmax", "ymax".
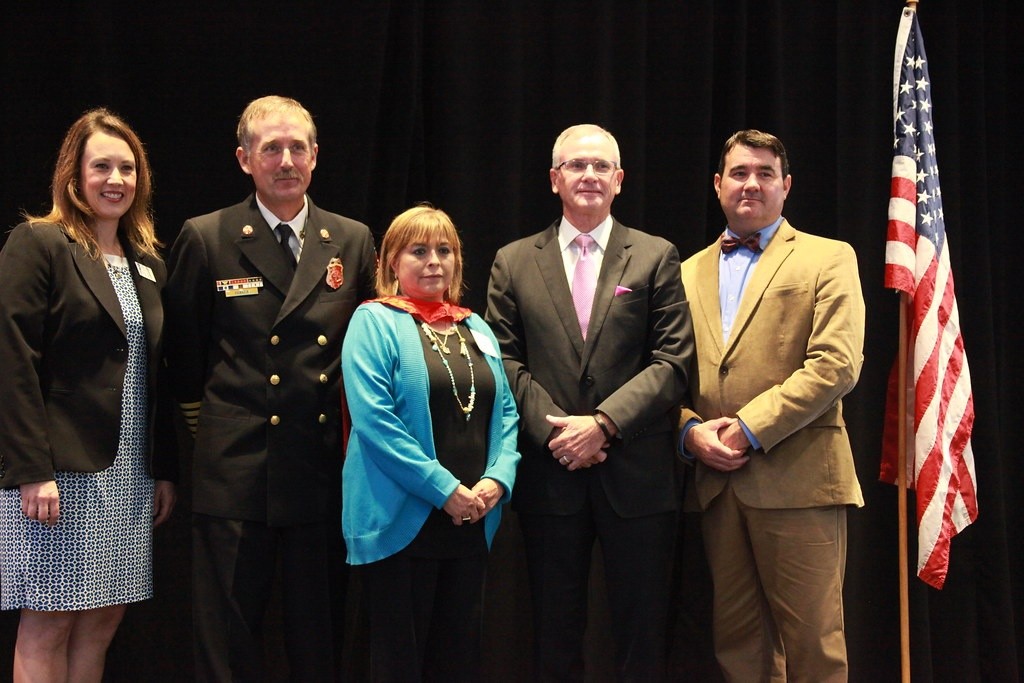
[
  {"xmin": 415, "ymin": 315, "xmax": 476, "ymax": 421},
  {"xmin": 104, "ymin": 247, "xmax": 124, "ymax": 280}
]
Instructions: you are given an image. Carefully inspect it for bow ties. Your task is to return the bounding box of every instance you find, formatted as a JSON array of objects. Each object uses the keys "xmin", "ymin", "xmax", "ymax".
[{"xmin": 721, "ymin": 232, "xmax": 762, "ymax": 254}]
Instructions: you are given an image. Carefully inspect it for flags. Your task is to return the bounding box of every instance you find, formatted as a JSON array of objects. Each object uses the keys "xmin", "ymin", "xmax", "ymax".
[{"xmin": 885, "ymin": 7, "xmax": 977, "ymax": 589}]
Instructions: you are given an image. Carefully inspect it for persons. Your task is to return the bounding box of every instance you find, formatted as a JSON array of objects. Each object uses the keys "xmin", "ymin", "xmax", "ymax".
[
  {"xmin": 0, "ymin": 109, "xmax": 180, "ymax": 683},
  {"xmin": 341, "ymin": 206, "xmax": 522, "ymax": 683},
  {"xmin": 166, "ymin": 96, "xmax": 378, "ymax": 683},
  {"xmin": 485, "ymin": 124, "xmax": 695, "ymax": 683},
  {"xmin": 677, "ymin": 129, "xmax": 865, "ymax": 683}
]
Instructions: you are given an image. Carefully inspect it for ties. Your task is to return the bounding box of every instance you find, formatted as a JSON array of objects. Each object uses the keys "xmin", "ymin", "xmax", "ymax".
[
  {"xmin": 572, "ymin": 233, "xmax": 596, "ymax": 342},
  {"xmin": 273, "ymin": 222, "xmax": 298, "ymax": 280}
]
